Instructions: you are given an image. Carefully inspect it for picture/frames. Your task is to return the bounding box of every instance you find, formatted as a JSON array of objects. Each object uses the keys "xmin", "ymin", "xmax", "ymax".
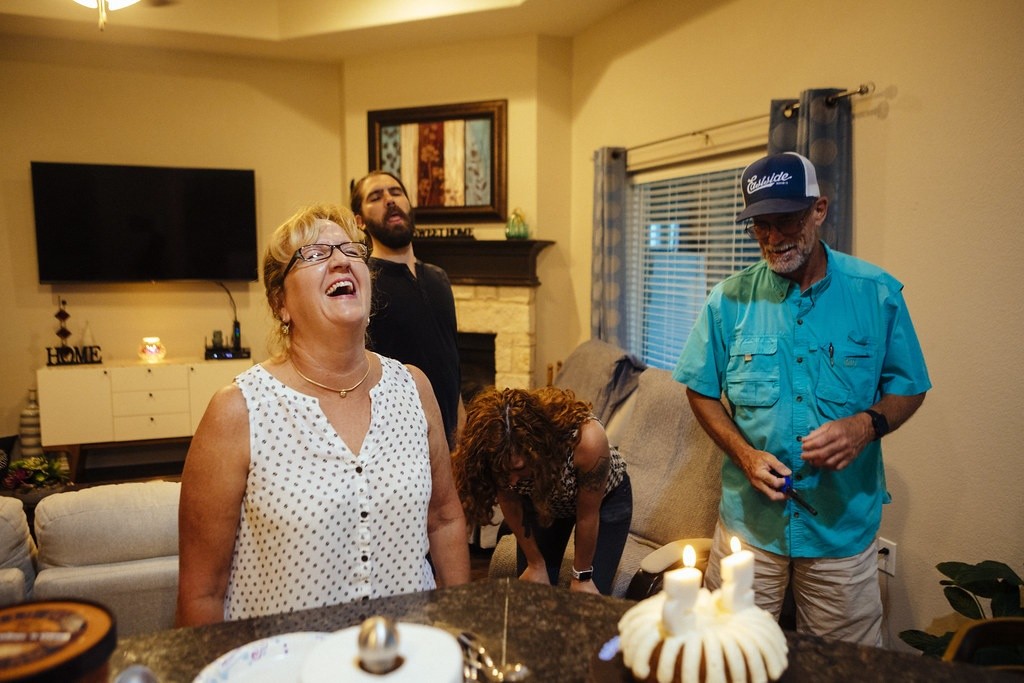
[{"xmin": 368, "ymin": 98, "xmax": 508, "ymax": 224}]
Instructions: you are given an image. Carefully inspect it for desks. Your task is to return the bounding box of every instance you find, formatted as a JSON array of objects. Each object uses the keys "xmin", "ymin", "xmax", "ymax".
[{"xmin": 72, "ymin": 577, "xmax": 1024, "ymax": 683}]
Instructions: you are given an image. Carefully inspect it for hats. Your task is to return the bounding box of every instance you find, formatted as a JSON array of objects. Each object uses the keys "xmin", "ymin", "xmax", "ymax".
[{"xmin": 735, "ymin": 151, "xmax": 821, "ymax": 222}]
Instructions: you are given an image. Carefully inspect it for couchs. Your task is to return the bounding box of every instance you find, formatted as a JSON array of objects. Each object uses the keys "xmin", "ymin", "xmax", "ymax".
[{"xmin": 0, "ymin": 478, "xmax": 182, "ymax": 638}]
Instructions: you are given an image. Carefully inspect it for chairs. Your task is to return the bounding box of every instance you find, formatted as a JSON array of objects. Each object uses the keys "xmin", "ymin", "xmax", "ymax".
[{"xmin": 942, "ymin": 617, "xmax": 1024, "ymax": 673}]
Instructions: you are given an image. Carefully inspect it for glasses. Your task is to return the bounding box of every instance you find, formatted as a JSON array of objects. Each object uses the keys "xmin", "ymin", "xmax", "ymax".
[
  {"xmin": 277, "ymin": 240, "xmax": 370, "ymax": 286},
  {"xmin": 745, "ymin": 204, "xmax": 814, "ymax": 239}
]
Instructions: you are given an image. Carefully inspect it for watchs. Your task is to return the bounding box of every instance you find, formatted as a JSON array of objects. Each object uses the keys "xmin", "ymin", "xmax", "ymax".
[
  {"xmin": 863, "ymin": 410, "xmax": 889, "ymax": 442},
  {"xmin": 571, "ymin": 565, "xmax": 593, "ymax": 580}
]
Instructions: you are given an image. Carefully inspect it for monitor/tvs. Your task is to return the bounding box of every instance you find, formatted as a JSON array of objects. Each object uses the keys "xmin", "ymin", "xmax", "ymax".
[{"xmin": 32, "ymin": 161, "xmax": 258, "ymax": 282}]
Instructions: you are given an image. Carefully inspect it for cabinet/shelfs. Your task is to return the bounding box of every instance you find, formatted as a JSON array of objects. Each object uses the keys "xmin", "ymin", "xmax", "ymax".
[{"xmin": 35, "ymin": 357, "xmax": 254, "ymax": 482}]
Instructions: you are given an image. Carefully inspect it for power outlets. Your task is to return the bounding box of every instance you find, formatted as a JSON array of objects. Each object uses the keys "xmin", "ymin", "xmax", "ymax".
[{"xmin": 877, "ymin": 537, "xmax": 897, "ymax": 578}]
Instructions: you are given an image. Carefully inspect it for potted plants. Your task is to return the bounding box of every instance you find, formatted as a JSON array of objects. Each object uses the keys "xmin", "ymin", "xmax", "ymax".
[{"xmin": 2, "ymin": 451, "xmax": 80, "ymax": 540}]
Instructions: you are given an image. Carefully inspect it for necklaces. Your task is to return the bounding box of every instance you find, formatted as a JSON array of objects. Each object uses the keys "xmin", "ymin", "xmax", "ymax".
[{"xmin": 289, "ymin": 355, "xmax": 370, "ymax": 397}]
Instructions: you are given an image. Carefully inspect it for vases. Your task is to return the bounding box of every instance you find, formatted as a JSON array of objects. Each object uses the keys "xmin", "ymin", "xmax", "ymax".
[{"xmin": 19, "ymin": 388, "xmax": 42, "ymax": 457}]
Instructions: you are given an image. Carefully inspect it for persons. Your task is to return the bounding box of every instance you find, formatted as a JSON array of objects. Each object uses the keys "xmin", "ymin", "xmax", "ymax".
[
  {"xmin": 174, "ymin": 201, "xmax": 471, "ymax": 628},
  {"xmin": 449, "ymin": 386, "xmax": 633, "ymax": 596},
  {"xmin": 350, "ymin": 171, "xmax": 461, "ymax": 455},
  {"xmin": 671, "ymin": 151, "xmax": 933, "ymax": 650}
]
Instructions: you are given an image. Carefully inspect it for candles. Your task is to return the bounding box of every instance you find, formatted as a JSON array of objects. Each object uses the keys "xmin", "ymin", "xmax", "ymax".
[
  {"xmin": 719, "ymin": 535, "xmax": 757, "ymax": 611},
  {"xmin": 661, "ymin": 543, "xmax": 705, "ymax": 637}
]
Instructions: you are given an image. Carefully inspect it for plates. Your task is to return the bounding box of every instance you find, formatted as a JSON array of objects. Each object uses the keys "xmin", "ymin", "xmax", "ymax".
[{"xmin": 193, "ymin": 632, "xmax": 337, "ymax": 683}]
